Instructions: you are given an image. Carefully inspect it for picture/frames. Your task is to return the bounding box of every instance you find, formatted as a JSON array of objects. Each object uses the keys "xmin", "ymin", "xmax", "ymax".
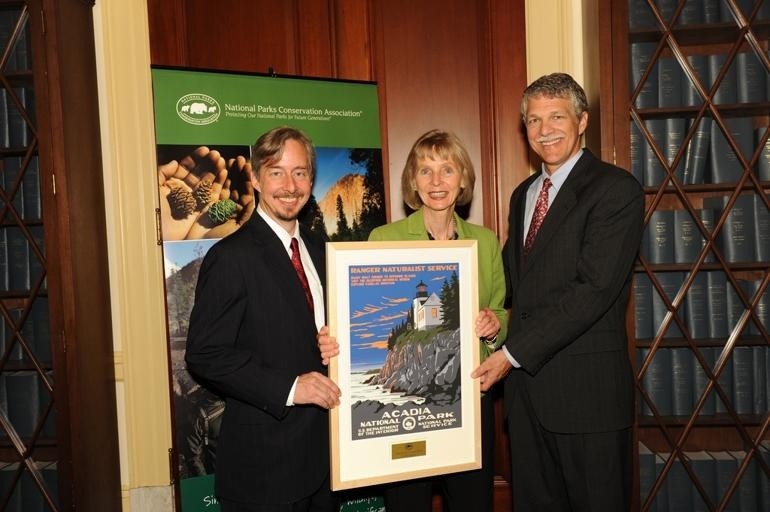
[{"xmin": 323, "ymin": 238, "xmax": 486, "ymax": 492}]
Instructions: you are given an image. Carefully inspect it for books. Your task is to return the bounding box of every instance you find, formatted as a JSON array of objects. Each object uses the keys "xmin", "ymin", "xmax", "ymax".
[
  {"xmin": 0, "ymin": 8, "xmax": 61, "ymax": 511},
  {"xmin": 629, "ymin": 0, "xmax": 770, "ymax": 512}
]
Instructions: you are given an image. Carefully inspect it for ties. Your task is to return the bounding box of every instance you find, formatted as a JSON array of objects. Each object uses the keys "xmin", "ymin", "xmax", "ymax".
[
  {"xmin": 525, "ymin": 178, "xmax": 553, "ymax": 255},
  {"xmin": 290, "ymin": 238, "xmax": 314, "ymax": 310}
]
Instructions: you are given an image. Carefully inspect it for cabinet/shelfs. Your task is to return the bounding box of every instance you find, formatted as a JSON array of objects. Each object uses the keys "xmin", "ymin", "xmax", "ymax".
[
  {"xmin": 610, "ymin": 0, "xmax": 769, "ymax": 512},
  {"xmin": 0, "ymin": 0, "xmax": 74, "ymax": 511}
]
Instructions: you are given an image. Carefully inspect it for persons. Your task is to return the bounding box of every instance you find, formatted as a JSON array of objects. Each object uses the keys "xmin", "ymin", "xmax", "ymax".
[
  {"xmin": 315, "ymin": 128, "xmax": 510, "ymax": 511},
  {"xmin": 471, "ymin": 73, "xmax": 644, "ymax": 511},
  {"xmin": 159, "ymin": 146, "xmax": 255, "ymax": 241},
  {"xmin": 185, "ymin": 126, "xmax": 341, "ymax": 512}
]
